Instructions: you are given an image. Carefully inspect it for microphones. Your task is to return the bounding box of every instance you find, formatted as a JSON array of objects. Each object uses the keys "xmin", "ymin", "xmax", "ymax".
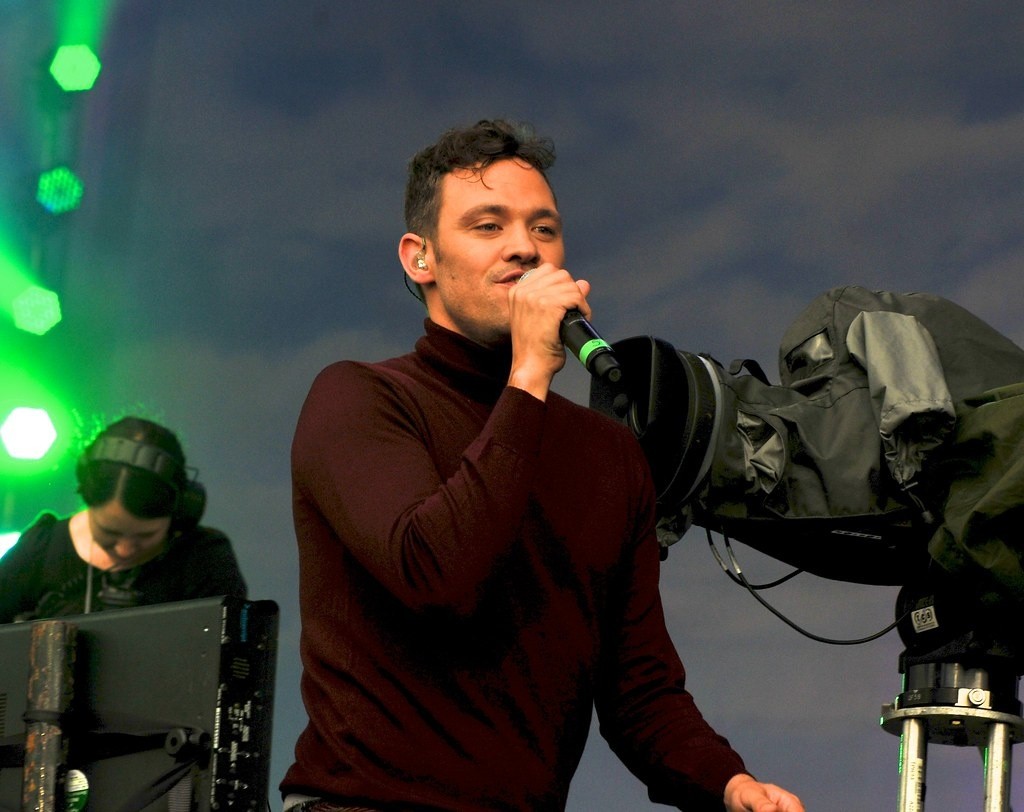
[{"xmin": 558, "ymin": 307, "xmax": 619, "ymax": 380}]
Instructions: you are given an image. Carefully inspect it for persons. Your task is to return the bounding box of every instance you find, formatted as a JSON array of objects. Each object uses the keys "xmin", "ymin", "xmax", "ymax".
[
  {"xmin": 277, "ymin": 119, "xmax": 808, "ymax": 812},
  {"xmin": 1, "ymin": 416, "xmax": 249, "ymax": 706}
]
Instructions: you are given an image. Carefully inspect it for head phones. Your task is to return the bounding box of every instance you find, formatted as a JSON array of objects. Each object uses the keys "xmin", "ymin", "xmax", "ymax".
[{"xmin": 78, "ymin": 438, "xmax": 207, "ymax": 532}]
